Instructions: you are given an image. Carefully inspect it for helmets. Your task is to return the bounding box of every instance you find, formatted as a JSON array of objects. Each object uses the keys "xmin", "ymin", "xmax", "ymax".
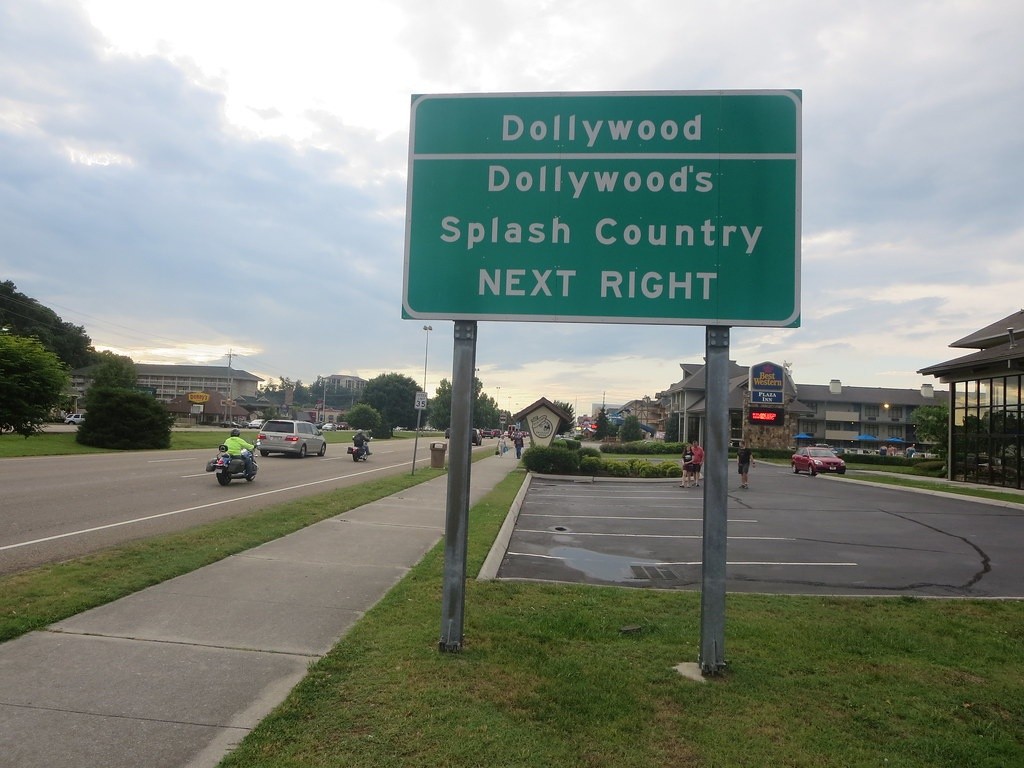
[
  {"xmin": 230, "ymin": 428, "xmax": 240, "ymax": 436},
  {"xmin": 357, "ymin": 429, "xmax": 363, "ymax": 434}
]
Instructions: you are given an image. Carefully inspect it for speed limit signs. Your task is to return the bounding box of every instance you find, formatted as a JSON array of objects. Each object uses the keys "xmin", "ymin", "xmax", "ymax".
[{"xmin": 414, "ymin": 391, "xmax": 428, "ymax": 411}]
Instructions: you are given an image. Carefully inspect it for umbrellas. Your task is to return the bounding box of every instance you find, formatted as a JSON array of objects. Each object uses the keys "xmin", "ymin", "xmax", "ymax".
[
  {"xmin": 884, "ymin": 437, "xmax": 906, "ymax": 444},
  {"xmin": 793, "ymin": 432, "xmax": 814, "ymax": 438},
  {"xmin": 852, "ymin": 433, "xmax": 881, "ymax": 440}
]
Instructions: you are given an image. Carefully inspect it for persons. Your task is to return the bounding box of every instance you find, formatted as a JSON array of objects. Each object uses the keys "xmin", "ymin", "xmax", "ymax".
[
  {"xmin": 514, "ymin": 434, "xmax": 524, "ymax": 459},
  {"xmin": 736, "ymin": 441, "xmax": 755, "ymax": 489},
  {"xmin": 222, "ymin": 428, "xmax": 257, "ymax": 475},
  {"xmin": 497, "ymin": 434, "xmax": 506, "ymax": 458},
  {"xmin": 352, "ymin": 429, "xmax": 373, "ymax": 455},
  {"xmin": 906, "ymin": 444, "xmax": 916, "ymax": 458},
  {"xmin": 679, "ymin": 439, "xmax": 703, "ymax": 488},
  {"xmin": 879, "ymin": 443, "xmax": 898, "ymax": 457}
]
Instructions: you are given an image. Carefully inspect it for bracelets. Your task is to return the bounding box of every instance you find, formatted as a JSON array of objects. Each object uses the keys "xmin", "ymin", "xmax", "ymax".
[{"xmin": 753, "ymin": 463, "xmax": 755, "ymax": 464}]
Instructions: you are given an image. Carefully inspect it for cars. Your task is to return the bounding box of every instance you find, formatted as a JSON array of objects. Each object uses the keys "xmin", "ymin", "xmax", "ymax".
[
  {"xmin": 482, "ymin": 428, "xmax": 530, "ymax": 441},
  {"xmin": 790, "ymin": 446, "xmax": 846, "ymax": 476},
  {"xmin": 256, "ymin": 419, "xmax": 326, "ymax": 458},
  {"xmin": 444, "ymin": 428, "xmax": 450, "ymax": 439},
  {"xmin": 248, "ymin": 419, "xmax": 267, "ymax": 429},
  {"xmin": 235, "ymin": 420, "xmax": 250, "ymax": 429},
  {"xmin": 220, "ymin": 421, "xmax": 243, "ymax": 429},
  {"xmin": 966, "ymin": 451, "xmax": 1001, "ymax": 467},
  {"xmin": 314, "ymin": 421, "xmax": 349, "ymax": 432},
  {"xmin": 471, "ymin": 427, "xmax": 482, "ymax": 446}
]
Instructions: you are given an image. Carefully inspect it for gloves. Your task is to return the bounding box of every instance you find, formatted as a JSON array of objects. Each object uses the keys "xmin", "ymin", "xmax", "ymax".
[{"xmin": 253, "ymin": 444, "xmax": 257, "ymax": 449}]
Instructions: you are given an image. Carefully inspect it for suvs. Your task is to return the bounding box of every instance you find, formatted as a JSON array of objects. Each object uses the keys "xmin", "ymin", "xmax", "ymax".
[{"xmin": 64, "ymin": 413, "xmax": 87, "ymax": 425}]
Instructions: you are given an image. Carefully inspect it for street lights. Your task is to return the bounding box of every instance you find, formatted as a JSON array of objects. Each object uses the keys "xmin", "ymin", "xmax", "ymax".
[{"xmin": 423, "ymin": 325, "xmax": 432, "ymax": 393}]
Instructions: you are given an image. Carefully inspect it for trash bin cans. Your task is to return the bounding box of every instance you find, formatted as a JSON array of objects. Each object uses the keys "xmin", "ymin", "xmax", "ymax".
[{"xmin": 430, "ymin": 441, "xmax": 447, "ymax": 470}]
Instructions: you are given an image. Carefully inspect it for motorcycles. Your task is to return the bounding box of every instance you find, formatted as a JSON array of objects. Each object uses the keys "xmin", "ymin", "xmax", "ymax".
[
  {"xmin": 205, "ymin": 441, "xmax": 261, "ymax": 486},
  {"xmin": 347, "ymin": 435, "xmax": 373, "ymax": 462}
]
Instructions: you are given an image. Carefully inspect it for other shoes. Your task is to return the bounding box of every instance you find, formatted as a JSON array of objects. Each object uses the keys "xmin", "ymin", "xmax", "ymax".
[
  {"xmin": 687, "ymin": 485, "xmax": 691, "ymax": 488},
  {"xmin": 366, "ymin": 452, "xmax": 373, "ymax": 455},
  {"xmin": 692, "ymin": 483, "xmax": 696, "ymax": 487},
  {"xmin": 246, "ymin": 471, "xmax": 256, "ymax": 479},
  {"xmin": 679, "ymin": 485, "xmax": 685, "ymax": 488},
  {"xmin": 738, "ymin": 482, "xmax": 748, "ymax": 489},
  {"xmin": 695, "ymin": 484, "xmax": 699, "ymax": 487}
]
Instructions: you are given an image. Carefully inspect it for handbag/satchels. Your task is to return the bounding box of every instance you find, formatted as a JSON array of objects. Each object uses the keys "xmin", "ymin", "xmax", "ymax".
[
  {"xmin": 503, "ymin": 447, "xmax": 508, "ymax": 455},
  {"xmin": 494, "ymin": 447, "xmax": 500, "ymax": 455}
]
